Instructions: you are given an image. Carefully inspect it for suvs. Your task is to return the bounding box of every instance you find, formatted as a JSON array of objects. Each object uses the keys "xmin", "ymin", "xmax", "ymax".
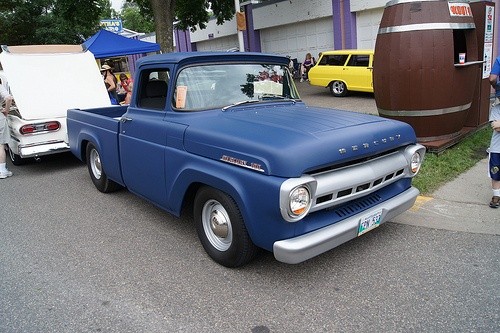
[{"xmin": 306, "ymin": 50, "xmax": 376, "ymax": 97}]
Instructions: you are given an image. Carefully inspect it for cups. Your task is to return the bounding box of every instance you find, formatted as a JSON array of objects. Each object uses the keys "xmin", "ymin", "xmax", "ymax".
[{"xmin": 459, "ymin": 53, "xmax": 466, "ymax": 64}]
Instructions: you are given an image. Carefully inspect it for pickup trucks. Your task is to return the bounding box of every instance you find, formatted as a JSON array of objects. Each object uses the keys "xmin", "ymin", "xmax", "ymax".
[{"xmin": 68, "ymin": 51, "xmax": 427, "ymax": 270}]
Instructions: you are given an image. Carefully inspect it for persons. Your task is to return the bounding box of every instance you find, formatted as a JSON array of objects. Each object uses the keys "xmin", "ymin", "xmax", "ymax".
[
  {"xmin": 489, "ymin": 55, "xmax": 500, "ymax": 208},
  {"xmin": 0, "ymin": 84, "xmax": 13, "ymax": 179},
  {"xmin": 99, "ymin": 65, "xmax": 134, "ymax": 105},
  {"xmin": 286, "ymin": 52, "xmax": 323, "ymax": 83}
]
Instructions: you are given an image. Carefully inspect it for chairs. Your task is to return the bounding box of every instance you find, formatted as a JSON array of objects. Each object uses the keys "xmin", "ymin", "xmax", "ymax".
[
  {"xmin": 291, "ymin": 57, "xmax": 316, "ymax": 79},
  {"xmin": 136, "ymin": 79, "xmax": 169, "ymax": 111}
]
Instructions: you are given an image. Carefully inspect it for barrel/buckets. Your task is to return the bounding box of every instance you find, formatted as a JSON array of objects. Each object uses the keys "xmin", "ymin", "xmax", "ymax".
[{"xmin": 372, "ymin": 0, "xmax": 479, "ymax": 142}]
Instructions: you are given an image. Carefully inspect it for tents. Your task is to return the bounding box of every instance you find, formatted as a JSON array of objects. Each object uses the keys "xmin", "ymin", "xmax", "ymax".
[{"xmin": 80, "ymin": 30, "xmax": 161, "ymax": 59}]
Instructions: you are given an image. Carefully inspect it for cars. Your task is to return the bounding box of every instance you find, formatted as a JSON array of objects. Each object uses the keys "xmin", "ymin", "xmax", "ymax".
[{"xmin": 0, "ymin": 44, "xmax": 112, "ymax": 167}]
